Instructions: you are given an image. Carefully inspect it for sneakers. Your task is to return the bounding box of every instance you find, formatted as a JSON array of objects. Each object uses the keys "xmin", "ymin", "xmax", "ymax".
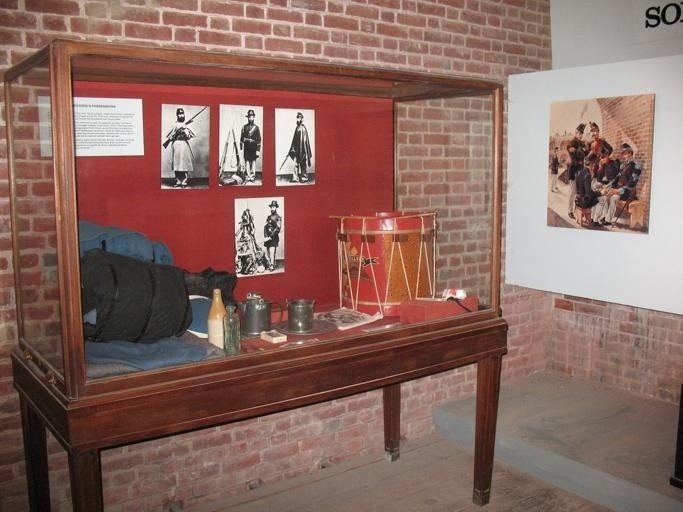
[
  {"xmin": 568, "ymin": 214, "xmax": 612, "ymax": 228},
  {"xmin": 173, "ymin": 184, "xmax": 185, "ymax": 189}
]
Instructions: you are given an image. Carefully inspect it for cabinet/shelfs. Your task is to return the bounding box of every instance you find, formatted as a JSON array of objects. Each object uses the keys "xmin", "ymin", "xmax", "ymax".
[{"xmin": 5, "ymin": 38, "xmax": 507, "ymax": 509}]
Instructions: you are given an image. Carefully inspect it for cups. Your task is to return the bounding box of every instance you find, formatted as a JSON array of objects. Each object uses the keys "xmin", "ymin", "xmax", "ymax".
[{"xmin": 288, "ymin": 299, "xmax": 314, "ymax": 333}]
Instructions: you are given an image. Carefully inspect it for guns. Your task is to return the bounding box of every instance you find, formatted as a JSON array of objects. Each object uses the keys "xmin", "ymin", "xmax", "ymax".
[
  {"xmin": 162, "ymin": 106, "xmax": 208, "ymax": 149},
  {"xmin": 232, "ymin": 128, "xmax": 242, "ymax": 177},
  {"xmin": 218, "ymin": 129, "xmax": 230, "ymax": 183}
]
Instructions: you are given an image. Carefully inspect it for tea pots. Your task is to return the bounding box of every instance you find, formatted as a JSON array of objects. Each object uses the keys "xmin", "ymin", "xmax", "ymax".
[{"xmin": 238, "ymin": 292, "xmax": 283, "ymax": 337}]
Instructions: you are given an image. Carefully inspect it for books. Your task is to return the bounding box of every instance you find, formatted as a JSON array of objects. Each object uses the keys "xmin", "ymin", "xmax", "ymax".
[{"xmin": 312, "ymin": 305, "xmax": 375, "ymax": 331}]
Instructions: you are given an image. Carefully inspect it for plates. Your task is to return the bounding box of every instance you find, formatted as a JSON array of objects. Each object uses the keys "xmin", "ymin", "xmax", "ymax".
[{"xmin": 276, "ymin": 318, "xmax": 337, "ymax": 336}]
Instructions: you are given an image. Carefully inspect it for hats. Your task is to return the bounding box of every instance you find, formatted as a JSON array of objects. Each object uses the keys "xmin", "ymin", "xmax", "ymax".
[
  {"xmin": 588, "ymin": 122, "xmax": 598, "ymax": 132},
  {"xmin": 245, "ymin": 110, "xmax": 255, "ymax": 118},
  {"xmin": 575, "ymin": 123, "xmax": 586, "ymax": 134},
  {"xmin": 620, "ymin": 143, "xmax": 633, "ymax": 155},
  {"xmin": 175, "ymin": 108, "xmax": 184, "ymax": 116},
  {"xmin": 584, "ymin": 155, "xmax": 596, "ymax": 162},
  {"xmin": 601, "ymin": 143, "xmax": 612, "ymax": 156},
  {"xmin": 267, "ymin": 201, "xmax": 278, "ymax": 208}
]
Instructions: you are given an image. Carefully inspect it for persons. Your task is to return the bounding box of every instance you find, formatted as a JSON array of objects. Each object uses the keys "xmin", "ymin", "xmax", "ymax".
[
  {"xmin": 549, "ymin": 121, "xmax": 641, "ymax": 227},
  {"xmin": 286, "ymin": 112, "xmax": 312, "ymax": 182},
  {"xmin": 239, "ymin": 109, "xmax": 260, "ymax": 182},
  {"xmin": 169, "ymin": 107, "xmax": 194, "ymax": 185},
  {"xmin": 264, "ymin": 201, "xmax": 281, "ymax": 271}
]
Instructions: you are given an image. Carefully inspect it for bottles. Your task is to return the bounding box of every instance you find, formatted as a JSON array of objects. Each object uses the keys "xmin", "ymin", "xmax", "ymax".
[
  {"xmin": 223, "ymin": 306, "xmax": 241, "ymax": 354},
  {"xmin": 207, "ymin": 288, "xmax": 227, "ymax": 350}
]
